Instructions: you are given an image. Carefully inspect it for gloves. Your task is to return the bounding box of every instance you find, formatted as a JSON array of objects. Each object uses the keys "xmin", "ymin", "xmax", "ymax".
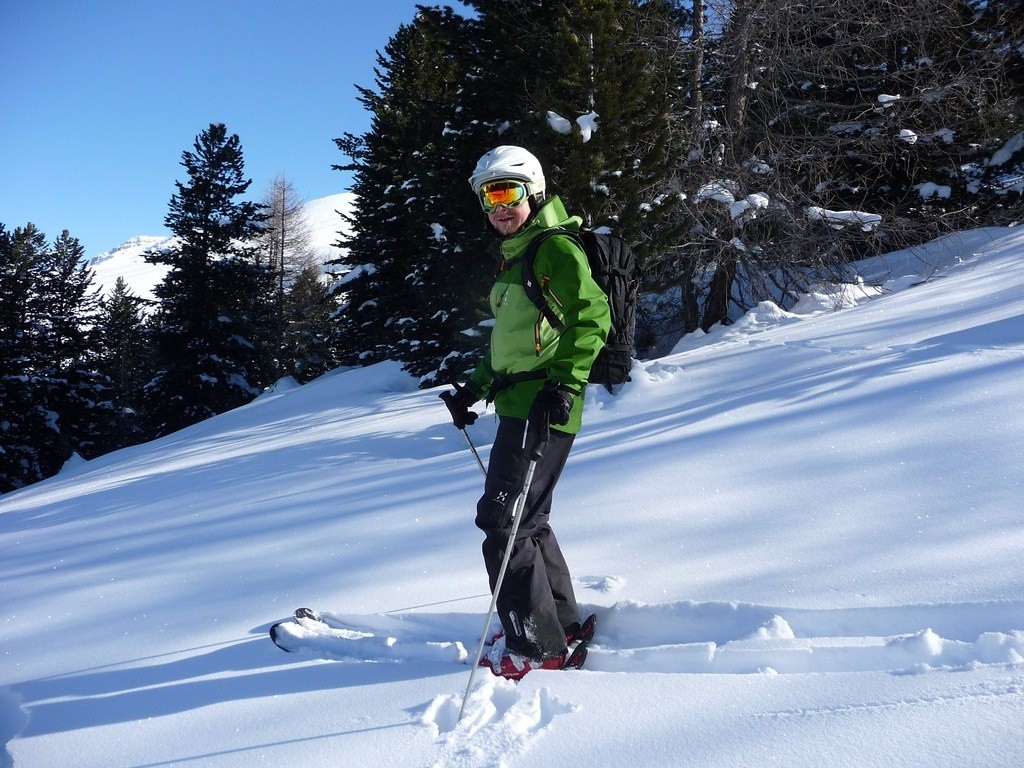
[
  {"xmin": 528, "ymin": 379, "xmax": 574, "ymax": 433},
  {"xmin": 446, "ymin": 382, "xmax": 478, "ymax": 430}
]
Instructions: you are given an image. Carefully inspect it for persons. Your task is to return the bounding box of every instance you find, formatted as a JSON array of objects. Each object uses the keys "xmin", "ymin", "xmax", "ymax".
[{"xmin": 454, "ymin": 145, "xmax": 613, "ymax": 680}]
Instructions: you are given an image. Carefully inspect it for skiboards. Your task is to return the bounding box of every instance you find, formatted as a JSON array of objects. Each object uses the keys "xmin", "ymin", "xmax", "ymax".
[{"xmin": 267, "ymin": 608, "xmax": 600, "ymax": 677}]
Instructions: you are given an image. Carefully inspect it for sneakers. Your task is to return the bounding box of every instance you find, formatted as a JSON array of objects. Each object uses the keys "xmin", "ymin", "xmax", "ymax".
[
  {"xmin": 492, "ymin": 622, "xmax": 581, "ymax": 647},
  {"xmin": 479, "ymin": 635, "xmax": 566, "ymax": 680}
]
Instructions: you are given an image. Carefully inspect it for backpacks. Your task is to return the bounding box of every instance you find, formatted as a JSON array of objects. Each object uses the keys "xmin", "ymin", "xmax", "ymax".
[{"xmin": 486, "ymin": 225, "xmax": 639, "ymax": 409}]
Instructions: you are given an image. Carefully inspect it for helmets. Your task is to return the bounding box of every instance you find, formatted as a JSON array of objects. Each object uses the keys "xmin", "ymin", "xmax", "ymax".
[{"xmin": 468, "ymin": 145, "xmax": 546, "ymax": 215}]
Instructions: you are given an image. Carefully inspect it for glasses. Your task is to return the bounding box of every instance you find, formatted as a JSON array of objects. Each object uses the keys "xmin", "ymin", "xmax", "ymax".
[{"xmin": 478, "ymin": 179, "xmax": 529, "ymax": 214}]
